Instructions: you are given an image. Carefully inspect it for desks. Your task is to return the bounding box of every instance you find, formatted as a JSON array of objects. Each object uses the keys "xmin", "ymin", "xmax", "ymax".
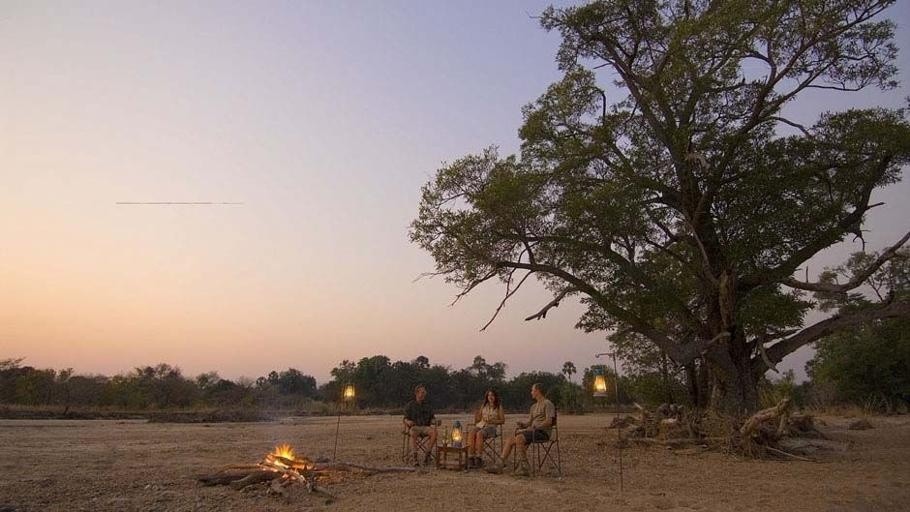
[{"xmin": 437, "ymin": 445, "xmax": 470, "ymax": 471}]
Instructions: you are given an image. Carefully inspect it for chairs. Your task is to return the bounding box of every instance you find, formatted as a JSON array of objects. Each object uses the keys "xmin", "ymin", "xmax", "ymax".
[
  {"xmin": 513, "ymin": 409, "xmax": 561, "ymax": 477},
  {"xmin": 466, "ymin": 420, "xmax": 503, "ymax": 464},
  {"xmin": 402, "ymin": 416, "xmax": 438, "ymax": 466}
]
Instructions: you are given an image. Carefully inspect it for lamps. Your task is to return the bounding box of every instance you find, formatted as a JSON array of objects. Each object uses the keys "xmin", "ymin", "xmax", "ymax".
[
  {"xmin": 334, "ymin": 383, "xmax": 356, "ymax": 460},
  {"xmin": 592, "ymin": 350, "xmax": 623, "ymax": 491},
  {"xmin": 450, "ymin": 421, "xmax": 463, "ymax": 447}
]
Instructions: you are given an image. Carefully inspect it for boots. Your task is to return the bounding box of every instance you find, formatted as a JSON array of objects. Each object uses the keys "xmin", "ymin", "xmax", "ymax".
[
  {"xmin": 510, "ymin": 467, "xmax": 529, "ymax": 475},
  {"xmin": 413, "ymin": 452, "xmax": 419, "ymax": 466},
  {"xmin": 467, "ymin": 456, "xmax": 481, "ymax": 468}
]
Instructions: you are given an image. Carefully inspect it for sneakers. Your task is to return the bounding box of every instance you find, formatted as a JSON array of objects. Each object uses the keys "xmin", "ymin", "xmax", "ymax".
[
  {"xmin": 423, "ymin": 452, "xmax": 432, "ymax": 466},
  {"xmin": 485, "ymin": 463, "xmax": 503, "ymax": 474}
]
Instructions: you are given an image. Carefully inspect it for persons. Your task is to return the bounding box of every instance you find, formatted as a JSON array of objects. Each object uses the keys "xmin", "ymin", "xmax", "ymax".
[
  {"xmin": 463, "ymin": 387, "xmax": 507, "ymax": 475},
  {"xmin": 401, "ymin": 385, "xmax": 444, "ymax": 467},
  {"xmin": 481, "ymin": 380, "xmax": 556, "ymax": 476}
]
